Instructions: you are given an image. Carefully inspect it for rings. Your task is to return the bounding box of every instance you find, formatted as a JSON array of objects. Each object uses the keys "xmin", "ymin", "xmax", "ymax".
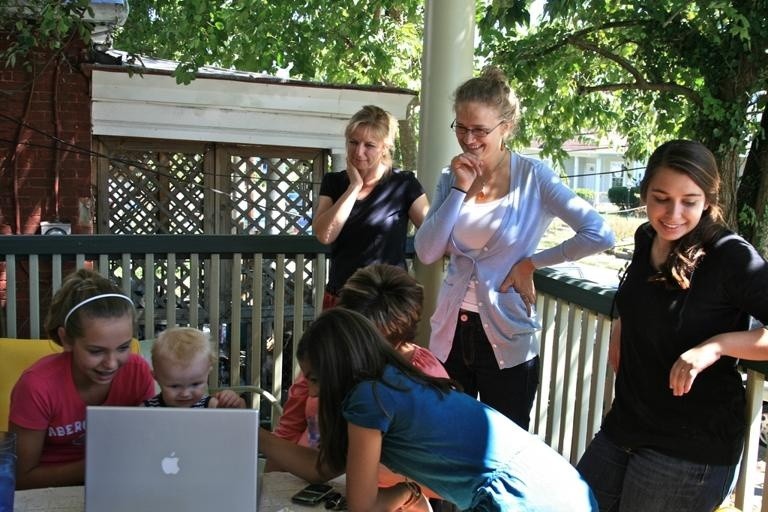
[{"xmin": 681, "ymin": 368, "xmax": 686, "ymax": 372}]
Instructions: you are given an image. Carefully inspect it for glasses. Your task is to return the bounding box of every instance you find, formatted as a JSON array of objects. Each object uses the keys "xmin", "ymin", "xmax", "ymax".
[{"xmin": 450, "ymin": 118, "xmax": 504, "ymax": 137}]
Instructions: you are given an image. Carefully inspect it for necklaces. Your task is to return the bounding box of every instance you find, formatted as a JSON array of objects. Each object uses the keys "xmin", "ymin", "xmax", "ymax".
[{"xmin": 478, "ymin": 150, "xmax": 507, "ymax": 199}]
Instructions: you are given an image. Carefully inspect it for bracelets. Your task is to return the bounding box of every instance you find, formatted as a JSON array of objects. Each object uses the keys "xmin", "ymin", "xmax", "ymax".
[
  {"xmin": 451, "ymin": 187, "xmax": 468, "ymax": 194},
  {"xmin": 399, "ymin": 480, "xmax": 421, "ymax": 510}
]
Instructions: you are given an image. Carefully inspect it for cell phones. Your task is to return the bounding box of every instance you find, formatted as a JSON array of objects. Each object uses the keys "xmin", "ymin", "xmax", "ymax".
[{"xmin": 291, "ymin": 483, "xmax": 335, "ymax": 504}]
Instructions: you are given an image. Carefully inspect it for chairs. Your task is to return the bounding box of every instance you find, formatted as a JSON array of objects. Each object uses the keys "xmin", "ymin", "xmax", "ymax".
[{"xmin": 0, "ymin": 332, "xmax": 141, "ymax": 442}]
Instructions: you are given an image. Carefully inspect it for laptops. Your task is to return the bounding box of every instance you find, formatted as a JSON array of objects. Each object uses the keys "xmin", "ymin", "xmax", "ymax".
[{"xmin": 82, "ymin": 405, "xmax": 267, "ymax": 511}]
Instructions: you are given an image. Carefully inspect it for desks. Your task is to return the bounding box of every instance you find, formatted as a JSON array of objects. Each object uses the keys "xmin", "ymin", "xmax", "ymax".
[{"xmin": 14, "ymin": 471, "xmax": 330, "ymax": 511}]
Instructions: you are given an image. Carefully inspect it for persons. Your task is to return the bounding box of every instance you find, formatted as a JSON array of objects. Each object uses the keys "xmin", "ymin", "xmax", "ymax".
[
  {"xmin": 9, "ymin": 268, "xmax": 155, "ymax": 490},
  {"xmin": 312, "ymin": 104, "xmax": 429, "ymax": 312},
  {"xmin": 242, "ymin": 258, "xmax": 449, "ymax": 485},
  {"xmin": 413, "ymin": 64, "xmax": 617, "ymax": 432},
  {"xmin": 139, "ymin": 328, "xmax": 240, "ymax": 407},
  {"xmin": 575, "ymin": 141, "xmax": 767, "ymax": 512},
  {"xmin": 296, "ymin": 307, "xmax": 600, "ymax": 511}
]
